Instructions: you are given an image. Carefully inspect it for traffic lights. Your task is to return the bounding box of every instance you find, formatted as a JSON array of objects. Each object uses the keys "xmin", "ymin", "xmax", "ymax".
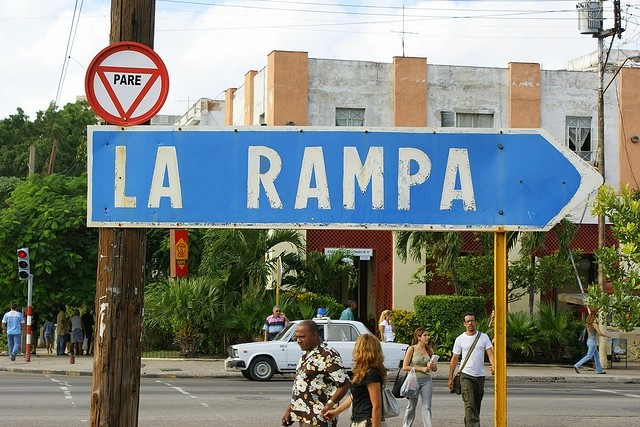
[{"xmin": 17, "ymin": 247, "xmax": 31, "ymax": 280}]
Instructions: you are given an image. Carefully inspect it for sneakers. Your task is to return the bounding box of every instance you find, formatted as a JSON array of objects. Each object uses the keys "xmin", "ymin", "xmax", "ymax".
[
  {"xmin": 598, "ymin": 372, "xmax": 606, "ymax": 374},
  {"xmin": 574, "ymin": 366, "xmax": 579, "ymax": 373},
  {"xmin": 11, "ymin": 354, "xmax": 15, "ymax": 361}
]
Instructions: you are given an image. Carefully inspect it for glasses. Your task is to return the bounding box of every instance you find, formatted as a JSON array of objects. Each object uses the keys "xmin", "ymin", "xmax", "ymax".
[{"xmin": 276, "ymin": 310, "xmax": 281, "ymax": 312}]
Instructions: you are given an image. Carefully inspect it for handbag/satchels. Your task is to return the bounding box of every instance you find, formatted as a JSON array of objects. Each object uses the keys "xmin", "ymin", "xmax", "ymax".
[
  {"xmin": 450, "ymin": 375, "xmax": 461, "ymax": 395},
  {"xmin": 391, "ymin": 375, "xmax": 407, "ymax": 399},
  {"xmin": 381, "ymin": 388, "xmax": 400, "ymax": 418},
  {"xmin": 578, "ymin": 327, "xmax": 588, "ymax": 346}
]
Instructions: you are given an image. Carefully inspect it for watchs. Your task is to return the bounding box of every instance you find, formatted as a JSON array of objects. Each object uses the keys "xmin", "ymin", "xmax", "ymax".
[{"xmin": 326, "ymin": 399, "xmax": 335, "ymax": 406}]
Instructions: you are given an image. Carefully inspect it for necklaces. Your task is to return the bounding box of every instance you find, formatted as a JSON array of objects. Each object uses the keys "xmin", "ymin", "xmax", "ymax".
[{"xmin": 417, "ymin": 344, "xmax": 426, "ymax": 356}]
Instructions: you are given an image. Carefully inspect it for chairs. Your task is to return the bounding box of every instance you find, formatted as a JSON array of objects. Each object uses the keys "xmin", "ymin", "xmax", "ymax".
[{"xmin": 607, "ymin": 338, "xmax": 628, "ymax": 369}]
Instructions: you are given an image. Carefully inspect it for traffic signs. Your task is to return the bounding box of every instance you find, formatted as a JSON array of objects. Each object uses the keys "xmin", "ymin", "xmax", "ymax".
[{"xmin": 86, "ymin": 125, "xmax": 605, "ymax": 233}]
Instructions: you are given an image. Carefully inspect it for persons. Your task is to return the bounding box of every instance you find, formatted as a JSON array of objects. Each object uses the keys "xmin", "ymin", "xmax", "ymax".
[
  {"xmin": 323, "ymin": 334, "xmax": 388, "ymax": 427},
  {"xmin": 282, "ymin": 320, "xmax": 350, "ymax": 427},
  {"xmin": 572, "ymin": 308, "xmax": 611, "ymax": 375},
  {"xmin": 447, "ymin": 312, "xmax": 495, "ymax": 427},
  {"xmin": 42, "ymin": 318, "xmax": 55, "ymax": 353},
  {"xmin": 1, "ymin": 302, "xmax": 25, "ymax": 363},
  {"xmin": 339, "ymin": 300, "xmax": 357, "ymax": 340},
  {"xmin": 262, "ymin": 305, "xmax": 290, "ymax": 341},
  {"xmin": 39, "ymin": 320, "xmax": 46, "ymax": 349},
  {"xmin": 23, "ymin": 306, "xmax": 39, "ymax": 355},
  {"xmin": 56, "ymin": 304, "xmax": 95, "ymax": 356},
  {"xmin": 378, "ymin": 308, "xmax": 397, "ymax": 343},
  {"xmin": 402, "ymin": 327, "xmax": 438, "ymax": 427},
  {"xmin": 488, "ymin": 308, "xmax": 496, "ymax": 378}
]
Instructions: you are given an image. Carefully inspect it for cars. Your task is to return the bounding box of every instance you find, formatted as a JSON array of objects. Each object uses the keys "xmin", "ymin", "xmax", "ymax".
[{"xmin": 223, "ymin": 307, "xmax": 411, "ymax": 382}]
